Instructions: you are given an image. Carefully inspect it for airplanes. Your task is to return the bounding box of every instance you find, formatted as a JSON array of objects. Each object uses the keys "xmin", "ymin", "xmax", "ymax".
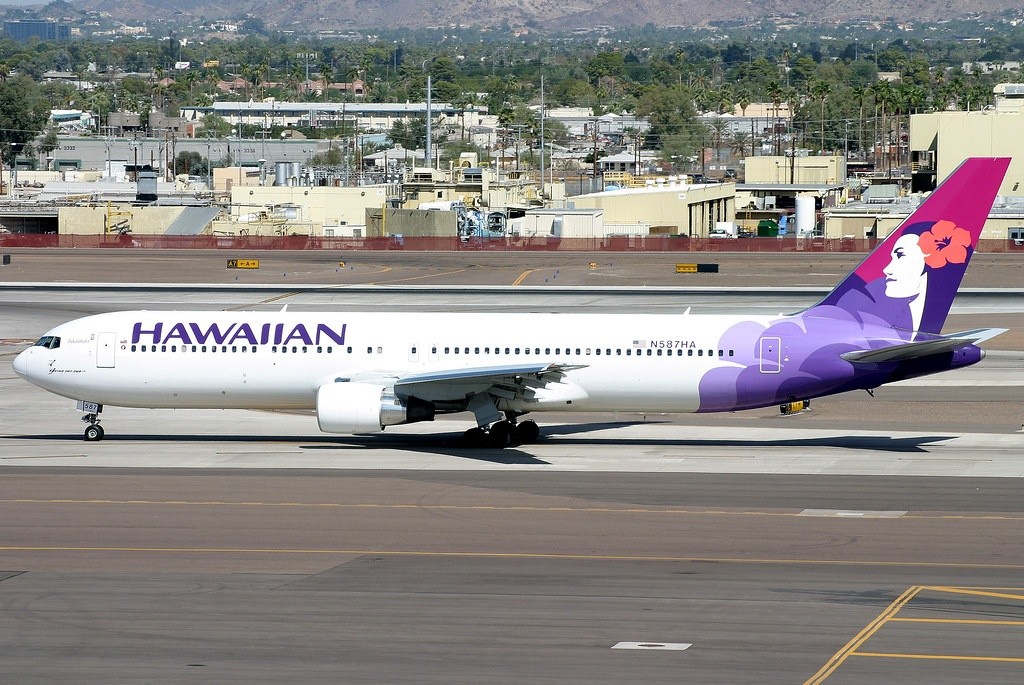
[{"xmin": 12, "ymin": 155, "xmax": 1014, "ymax": 452}]
